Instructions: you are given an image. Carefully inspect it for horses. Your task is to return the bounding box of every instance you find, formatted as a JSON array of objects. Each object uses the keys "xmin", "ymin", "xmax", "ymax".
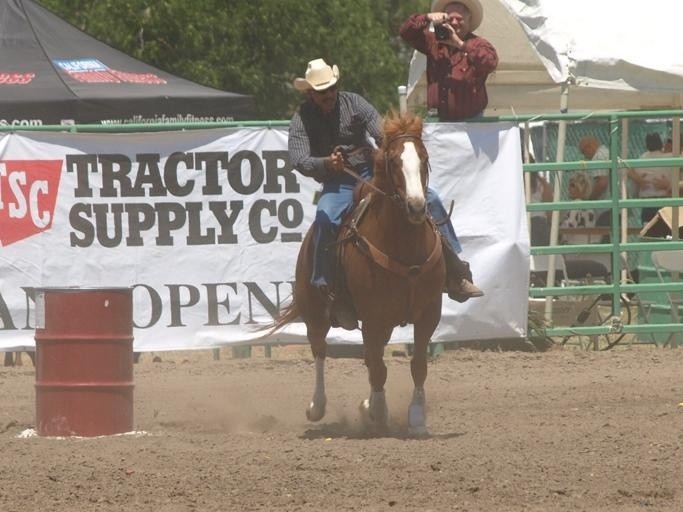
[{"xmin": 247, "ymin": 102, "xmax": 447, "ymax": 438}]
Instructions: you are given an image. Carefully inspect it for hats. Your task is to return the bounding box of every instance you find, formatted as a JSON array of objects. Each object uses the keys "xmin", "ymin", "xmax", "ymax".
[
  {"xmin": 294, "ymin": 58, "xmax": 339, "ymax": 92},
  {"xmin": 431, "ymin": 0, "xmax": 483, "ymax": 33}
]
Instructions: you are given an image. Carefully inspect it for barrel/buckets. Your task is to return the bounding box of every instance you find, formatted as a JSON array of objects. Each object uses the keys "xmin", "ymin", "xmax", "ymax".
[{"xmin": 32, "ymin": 287, "xmax": 134, "ymax": 439}]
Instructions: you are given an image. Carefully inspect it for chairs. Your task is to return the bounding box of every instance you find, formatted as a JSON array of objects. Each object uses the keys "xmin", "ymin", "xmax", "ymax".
[
  {"xmin": 558, "ymin": 197, "xmax": 603, "ymax": 244},
  {"xmin": 651, "ymin": 250, "xmax": 683, "ymax": 348},
  {"xmin": 530, "ymin": 254, "xmax": 583, "ymax": 350},
  {"xmin": 578, "ymin": 244, "xmax": 659, "ymax": 347},
  {"xmin": 531, "ymin": 216, "xmax": 607, "ymax": 350}
]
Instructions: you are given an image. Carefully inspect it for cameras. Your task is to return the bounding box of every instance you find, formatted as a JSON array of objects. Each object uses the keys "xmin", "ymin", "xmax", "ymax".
[{"xmin": 434, "ymin": 23, "xmax": 450, "ymax": 40}]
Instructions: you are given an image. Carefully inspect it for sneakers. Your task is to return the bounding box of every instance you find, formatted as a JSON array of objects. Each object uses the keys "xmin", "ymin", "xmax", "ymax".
[
  {"xmin": 443, "ymin": 277, "xmax": 483, "ymax": 298},
  {"xmin": 321, "ymin": 286, "xmax": 358, "ymax": 330}
]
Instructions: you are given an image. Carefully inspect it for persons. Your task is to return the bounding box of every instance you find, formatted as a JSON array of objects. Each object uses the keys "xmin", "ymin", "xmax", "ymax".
[
  {"xmin": 627, "ymin": 138, "xmax": 683, "ymax": 239},
  {"xmin": 559, "ymin": 170, "xmax": 597, "ymax": 228},
  {"xmin": 579, "ymin": 135, "xmax": 639, "ymax": 301},
  {"xmin": 287, "ymin": 58, "xmax": 484, "ymax": 299},
  {"xmin": 630, "ymin": 132, "xmax": 673, "ymax": 237},
  {"xmin": 399, "ymin": 0, "xmax": 498, "ymax": 123},
  {"xmin": 521, "ymin": 150, "xmax": 559, "ymax": 298}
]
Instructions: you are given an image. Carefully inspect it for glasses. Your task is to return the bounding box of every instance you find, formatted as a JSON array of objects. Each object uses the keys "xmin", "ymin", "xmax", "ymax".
[{"xmin": 314, "ymin": 85, "xmax": 337, "ymax": 94}]
